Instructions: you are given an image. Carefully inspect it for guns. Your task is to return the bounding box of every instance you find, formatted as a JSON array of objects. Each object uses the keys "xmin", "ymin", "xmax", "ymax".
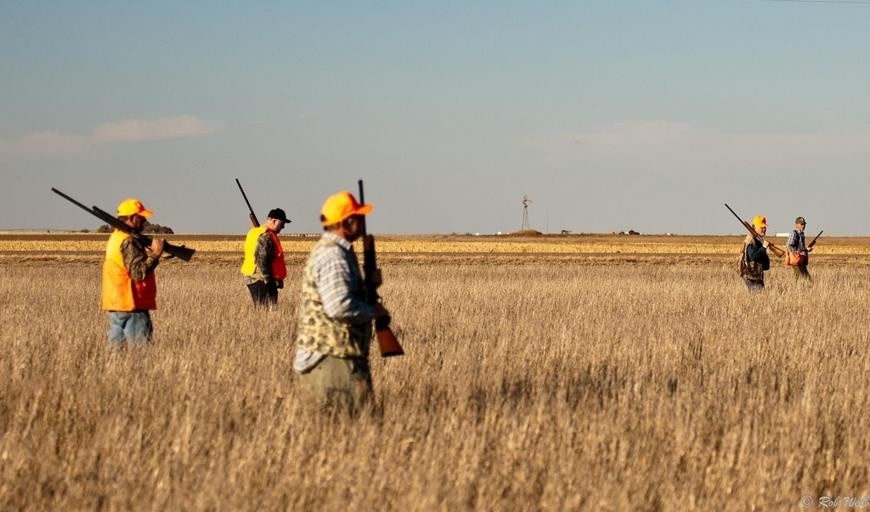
[
  {"xmin": 724, "ymin": 203, "xmax": 786, "ymax": 258},
  {"xmin": 52, "ymin": 185, "xmax": 196, "ymax": 261},
  {"xmin": 358, "ymin": 178, "xmax": 405, "ymax": 358},
  {"xmin": 235, "ymin": 178, "xmax": 284, "ymax": 291},
  {"xmin": 805, "ymin": 230, "xmax": 823, "ymax": 250}
]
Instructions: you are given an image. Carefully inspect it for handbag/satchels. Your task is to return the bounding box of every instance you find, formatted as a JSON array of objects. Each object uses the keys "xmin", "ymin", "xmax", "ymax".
[{"xmin": 786, "ymin": 247, "xmax": 808, "ymax": 266}]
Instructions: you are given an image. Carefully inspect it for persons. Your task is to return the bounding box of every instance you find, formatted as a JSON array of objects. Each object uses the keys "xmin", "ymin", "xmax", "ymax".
[
  {"xmin": 785, "ymin": 216, "xmax": 814, "ymax": 288},
  {"xmin": 241, "ymin": 208, "xmax": 291, "ymax": 311},
  {"xmin": 735, "ymin": 215, "xmax": 770, "ymax": 294},
  {"xmin": 101, "ymin": 198, "xmax": 166, "ymax": 351},
  {"xmin": 291, "ymin": 190, "xmax": 392, "ymax": 430}
]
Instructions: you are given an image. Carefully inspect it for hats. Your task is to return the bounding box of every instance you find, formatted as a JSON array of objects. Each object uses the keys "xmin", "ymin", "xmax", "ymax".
[
  {"xmin": 268, "ymin": 208, "xmax": 291, "ymax": 223},
  {"xmin": 117, "ymin": 199, "xmax": 154, "ymax": 218},
  {"xmin": 751, "ymin": 215, "xmax": 768, "ymax": 228},
  {"xmin": 795, "ymin": 217, "xmax": 807, "ymax": 225},
  {"xmin": 320, "ymin": 191, "xmax": 374, "ymax": 226}
]
